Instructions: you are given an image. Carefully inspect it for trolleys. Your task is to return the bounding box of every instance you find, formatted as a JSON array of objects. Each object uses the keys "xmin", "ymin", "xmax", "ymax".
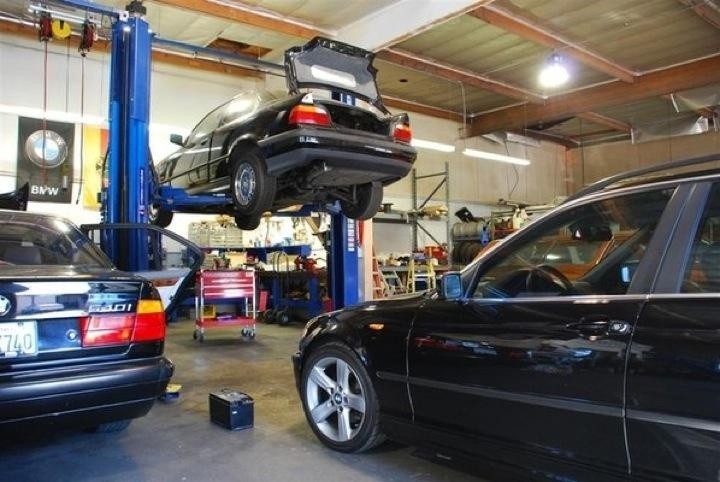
[{"xmin": 193, "ymin": 263, "xmax": 256, "ymax": 342}]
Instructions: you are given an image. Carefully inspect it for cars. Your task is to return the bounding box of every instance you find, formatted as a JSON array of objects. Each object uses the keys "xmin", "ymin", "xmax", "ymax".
[
  {"xmin": 148, "ymin": 36, "xmax": 418, "ymax": 231},
  {"xmin": 0, "ymin": 211, "xmax": 205, "ymax": 438},
  {"xmin": 291, "ymin": 153, "xmax": 720, "ymax": 482}
]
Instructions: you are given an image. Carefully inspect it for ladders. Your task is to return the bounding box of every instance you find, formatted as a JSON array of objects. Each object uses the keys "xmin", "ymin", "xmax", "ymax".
[
  {"xmin": 406, "ymin": 255, "xmax": 437, "ymax": 295},
  {"xmin": 372, "ymin": 246, "xmax": 384, "ymax": 299}
]
